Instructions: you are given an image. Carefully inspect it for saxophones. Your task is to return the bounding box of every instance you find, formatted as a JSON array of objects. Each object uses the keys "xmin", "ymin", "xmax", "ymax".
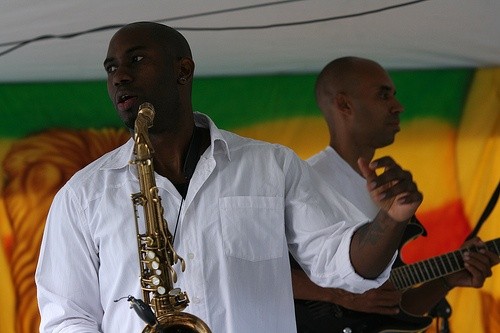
[{"xmin": 111, "ymin": 100, "xmax": 214, "ymax": 333}]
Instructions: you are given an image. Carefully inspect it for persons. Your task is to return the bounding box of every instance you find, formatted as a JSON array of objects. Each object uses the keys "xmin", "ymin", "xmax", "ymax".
[
  {"xmin": 33, "ymin": 19, "xmax": 425, "ymax": 333},
  {"xmin": 280, "ymin": 54, "xmax": 494, "ymax": 333}
]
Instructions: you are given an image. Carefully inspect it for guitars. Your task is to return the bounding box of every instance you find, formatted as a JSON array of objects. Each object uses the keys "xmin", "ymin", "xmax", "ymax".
[{"xmin": 288, "ymin": 223, "xmax": 499, "ymax": 333}]
